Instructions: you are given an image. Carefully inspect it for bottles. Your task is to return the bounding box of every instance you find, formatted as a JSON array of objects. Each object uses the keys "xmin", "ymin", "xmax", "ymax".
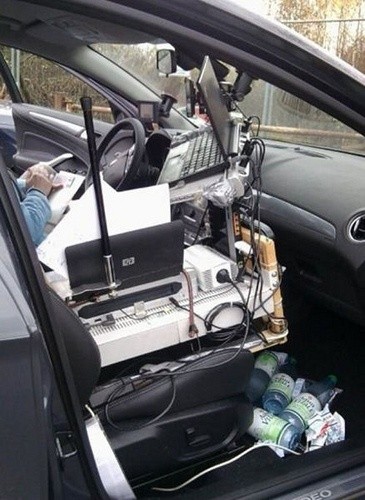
[
  {"xmin": 247, "ymin": 350, "xmax": 279, "ymax": 400},
  {"xmin": 262, "ymin": 358, "xmax": 297, "ymax": 414},
  {"xmin": 237, "ymin": 404, "xmax": 306, "ymax": 453},
  {"xmin": 279, "ymin": 375, "xmax": 337, "ymax": 433}
]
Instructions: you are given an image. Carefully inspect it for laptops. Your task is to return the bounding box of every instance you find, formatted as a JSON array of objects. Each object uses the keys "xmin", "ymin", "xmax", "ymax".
[
  {"xmin": 156, "ymin": 55, "xmax": 241, "ymax": 187},
  {"xmin": 64, "ymin": 220, "xmax": 186, "ymax": 302}
]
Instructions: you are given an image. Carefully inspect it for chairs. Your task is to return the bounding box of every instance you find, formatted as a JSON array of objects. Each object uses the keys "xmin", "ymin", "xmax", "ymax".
[{"xmin": 44, "ymin": 282, "xmax": 256, "ymax": 491}]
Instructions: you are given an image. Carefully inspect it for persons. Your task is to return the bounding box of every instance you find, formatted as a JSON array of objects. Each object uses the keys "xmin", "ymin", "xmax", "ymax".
[{"xmin": 4, "ymin": 166, "xmax": 63, "ymax": 245}]
